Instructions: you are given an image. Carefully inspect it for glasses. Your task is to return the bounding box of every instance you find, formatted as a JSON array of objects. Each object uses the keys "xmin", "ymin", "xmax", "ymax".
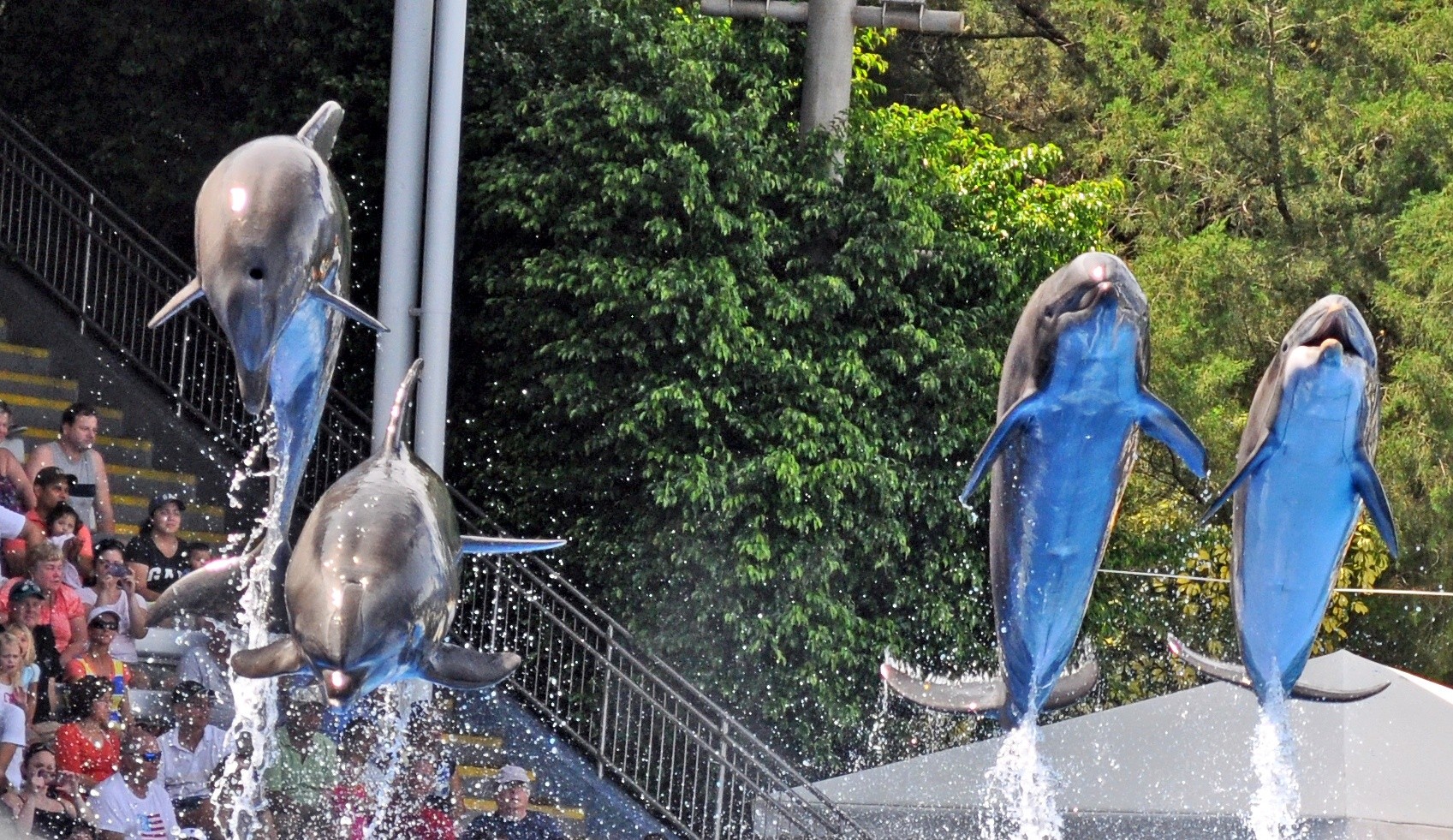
[
  {"xmin": 128, "ymin": 751, "xmax": 161, "ymax": 763},
  {"xmin": 91, "ymin": 620, "xmax": 119, "ymax": 631},
  {"xmin": 10, "ymin": 584, "xmax": 42, "ymax": 594},
  {"xmin": 25, "ymin": 743, "xmax": 56, "ymax": 755},
  {"xmin": 94, "ymin": 539, "xmax": 125, "ymax": 550}
]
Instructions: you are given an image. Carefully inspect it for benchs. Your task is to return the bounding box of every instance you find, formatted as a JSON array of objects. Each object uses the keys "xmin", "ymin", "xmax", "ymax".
[{"xmin": 0, "ymin": 319, "xmax": 587, "ymax": 840}]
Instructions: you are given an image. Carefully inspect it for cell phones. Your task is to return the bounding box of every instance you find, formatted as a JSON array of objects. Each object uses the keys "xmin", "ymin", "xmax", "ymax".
[{"xmin": 39, "ymin": 771, "xmax": 68, "ymax": 787}]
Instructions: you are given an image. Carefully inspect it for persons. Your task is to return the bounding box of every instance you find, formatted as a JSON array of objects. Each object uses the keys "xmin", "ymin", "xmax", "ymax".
[
  {"xmin": 0, "ymin": 403, "xmax": 464, "ymax": 840},
  {"xmin": 460, "ymin": 766, "xmax": 561, "ymax": 840}
]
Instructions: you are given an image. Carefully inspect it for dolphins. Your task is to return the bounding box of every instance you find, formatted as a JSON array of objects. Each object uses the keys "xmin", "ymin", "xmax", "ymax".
[
  {"xmin": 879, "ymin": 252, "xmax": 1205, "ymax": 731},
  {"xmin": 230, "ymin": 357, "xmax": 567, "ymax": 714},
  {"xmin": 142, "ymin": 100, "xmax": 392, "ymax": 638},
  {"xmin": 1167, "ymin": 294, "xmax": 1397, "ymax": 710}
]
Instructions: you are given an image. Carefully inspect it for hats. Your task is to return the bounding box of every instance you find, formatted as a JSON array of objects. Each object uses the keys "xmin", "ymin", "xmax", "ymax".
[
  {"xmin": 170, "ymin": 680, "xmax": 215, "ymax": 705},
  {"xmin": 34, "ymin": 466, "xmax": 78, "ymax": 490},
  {"xmin": 148, "ymin": 491, "xmax": 187, "ymax": 516},
  {"xmin": 87, "ymin": 606, "xmax": 122, "ymax": 629},
  {"xmin": 8, "ymin": 579, "xmax": 46, "ymax": 603},
  {"xmin": 288, "ymin": 686, "xmax": 327, "ymax": 715},
  {"xmin": 494, "ymin": 765, "xmax": 530, "ymax": 785}
]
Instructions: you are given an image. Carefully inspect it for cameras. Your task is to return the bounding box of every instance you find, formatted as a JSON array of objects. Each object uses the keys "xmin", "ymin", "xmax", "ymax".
[{"xmin": 106, "ymin": 562, "xmax": 130, "ymax": 577}]
